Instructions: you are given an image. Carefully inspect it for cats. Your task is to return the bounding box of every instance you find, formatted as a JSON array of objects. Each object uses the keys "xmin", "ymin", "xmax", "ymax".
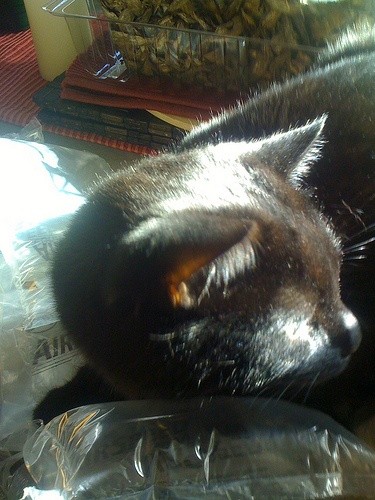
[{"xmin": 32, "ymin": 18, "xmax": 372, "ymax": 456}]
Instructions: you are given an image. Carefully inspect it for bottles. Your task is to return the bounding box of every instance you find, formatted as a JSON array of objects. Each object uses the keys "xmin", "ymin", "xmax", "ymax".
[{"xmin": 23, "ymin": 0, "xmax": 96, "ymax": 81}]
